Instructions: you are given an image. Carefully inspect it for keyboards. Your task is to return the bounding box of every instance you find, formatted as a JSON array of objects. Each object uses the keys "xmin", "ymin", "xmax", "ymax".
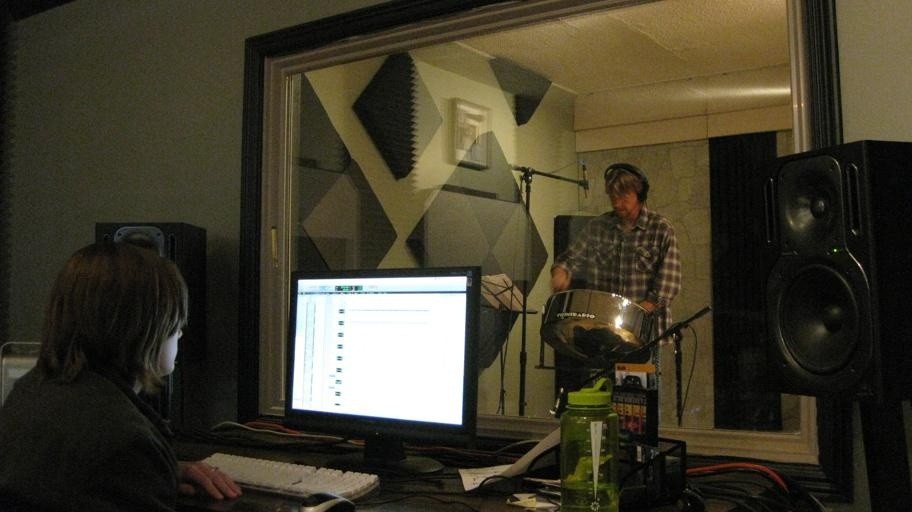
[{"xmin": 201, "ymin": 452, "xmax": 380, "ymax": 500}]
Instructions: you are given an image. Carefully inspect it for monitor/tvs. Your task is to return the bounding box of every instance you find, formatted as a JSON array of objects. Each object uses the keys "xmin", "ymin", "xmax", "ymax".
[{"xmin": 281, "ymin": 263, "xmax": 483, "ymax": 473}]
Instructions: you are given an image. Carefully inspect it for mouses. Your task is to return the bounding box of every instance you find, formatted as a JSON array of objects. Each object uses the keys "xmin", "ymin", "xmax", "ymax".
[{"xmin": 298, "ymin": 491, "xmax": 356, "ymax": 512}]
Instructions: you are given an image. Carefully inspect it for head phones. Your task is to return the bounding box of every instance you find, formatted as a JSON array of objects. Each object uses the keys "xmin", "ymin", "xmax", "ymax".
[{"xmin": 604, "ymin": 163, "xmax": 649, "ymax": 201}]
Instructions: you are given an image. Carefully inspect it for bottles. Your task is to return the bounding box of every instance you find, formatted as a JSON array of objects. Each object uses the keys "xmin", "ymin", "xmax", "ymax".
[{"xmin": 560, "ymin": 378, "xmax": 620, "ymax": 512}]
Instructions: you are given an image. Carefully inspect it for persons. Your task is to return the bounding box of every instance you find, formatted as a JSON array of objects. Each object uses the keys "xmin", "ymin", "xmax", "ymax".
[
  {"xmin": 0, "ymin": 243, "xmax": 243, "ymax": 511},
  {"xmin": 547, "ymin": 163, "xmax": 680, "ymax": 347}
]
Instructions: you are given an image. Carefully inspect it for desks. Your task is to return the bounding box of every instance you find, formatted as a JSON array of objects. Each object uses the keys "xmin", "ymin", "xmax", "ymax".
[{"xmin": 175, "ymin": 431, "xmax": 782, "ymax": 512}]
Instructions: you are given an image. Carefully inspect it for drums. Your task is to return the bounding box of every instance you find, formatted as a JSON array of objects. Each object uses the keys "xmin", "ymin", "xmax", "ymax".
[{"xmin": 542, "ymin": 290, "xmax": 655, "ymax": 364}]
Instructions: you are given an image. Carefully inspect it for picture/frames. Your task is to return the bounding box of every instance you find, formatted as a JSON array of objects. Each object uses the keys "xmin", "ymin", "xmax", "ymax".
[{"xmin": 448, "ymin": 97, "xmax": 492, "ymax": 170}]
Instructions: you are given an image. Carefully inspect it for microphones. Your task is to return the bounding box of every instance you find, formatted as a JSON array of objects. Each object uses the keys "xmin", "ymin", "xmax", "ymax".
[{"xmin": 582, "ymin": 164, "xmax": 591, "ymax": 199}]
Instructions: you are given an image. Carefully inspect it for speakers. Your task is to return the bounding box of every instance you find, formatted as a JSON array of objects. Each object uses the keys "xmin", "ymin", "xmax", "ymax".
[
  {"xmin": 759, "ymin": 140, "xmax": 912, "ymax": 404},
  {"xmin": 95, "ymin": 222, "xmax": 207, "ymax": 362}
]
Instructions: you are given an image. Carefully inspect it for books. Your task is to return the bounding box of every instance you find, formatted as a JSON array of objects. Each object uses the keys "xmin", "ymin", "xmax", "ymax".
[{"xmin": 610, "ymin": 361, "xmax": 659, "ymax": 449}]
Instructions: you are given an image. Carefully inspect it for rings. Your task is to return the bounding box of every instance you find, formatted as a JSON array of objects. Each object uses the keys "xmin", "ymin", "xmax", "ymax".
[{"xmin": 211, "ymin": 467, "xmax": 217, "ymax": 472}]
